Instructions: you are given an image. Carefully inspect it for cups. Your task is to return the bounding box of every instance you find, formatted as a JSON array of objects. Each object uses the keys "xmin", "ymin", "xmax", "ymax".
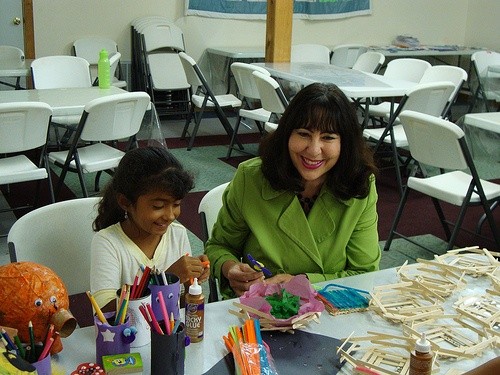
[
  {"xmin": 149, "ymin": 319, "xmax": 186, "ymax": 375},
  {"xmin": 149, "ymin": 273, "xmax": 181, "ymax": 322},
  {"xmin": 93, "ymin": 311, "xmax": 130, "ymax": 366},
  {"xmin": 116, "ymin": 285, "xmax": 152, "ymax": 348},
  {"xmin": 20, "ymin": 344, "xmax": 53, "ymax": 374}
]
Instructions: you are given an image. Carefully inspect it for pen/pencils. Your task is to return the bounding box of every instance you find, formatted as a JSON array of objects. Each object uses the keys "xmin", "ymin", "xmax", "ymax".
[
  {"xmin": 113, "ymin": 265, "xmax": 182, "ymax": 335},
  {"xmin": 86, "ymin": 291, "xmax": 107, "ymax": 323},
  {"xmin": 0, "ymin": 320, "xmax": 59, "ymax": 362}
]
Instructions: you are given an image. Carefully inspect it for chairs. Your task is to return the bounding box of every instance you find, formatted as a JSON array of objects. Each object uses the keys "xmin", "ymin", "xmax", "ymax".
[{"xmin": 0, "ymin": 16, "xmax": 500, "ymax": 328}]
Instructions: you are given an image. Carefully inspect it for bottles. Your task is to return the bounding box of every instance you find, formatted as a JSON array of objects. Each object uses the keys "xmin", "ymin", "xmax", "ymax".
[
  {"xmin": 98, "ymin": 48, "xmax": 110, "ymax": 89},
  {"xmin": 185, "ymin": 277, "xmax": 205, "ymax": 343},
  {"xmin": 409, "ymin": 332, "xmax": 432, "ymax": 375}
]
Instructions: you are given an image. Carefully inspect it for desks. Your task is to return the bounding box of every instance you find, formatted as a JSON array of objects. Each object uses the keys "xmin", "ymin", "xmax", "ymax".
[
  {"xmin": 249, "ymin": 59, "xmax": 424, "ymax": 197},
  {"xmin": 206, "ymin": 45, "xmax": 265, "ymax": 96},
  {"xmin": 50, "ymin": 250, "xmax": 500, "ymax": 375},
  {"xmin": 463, "ymin": 110, "xmax": 500, "ymax": 139},
  {"xmin": 372, "ymin": 42, "xmax": 482, "ymax": 108},
  {"xmin": 0, "ymin": 87, "xmax": 152, "ymax": 149}
]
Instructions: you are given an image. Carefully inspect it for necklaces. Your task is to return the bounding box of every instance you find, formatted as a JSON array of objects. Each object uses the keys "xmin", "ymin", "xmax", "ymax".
[{"xmin": 296, "ymin": 182, "xmax": 323, "ymax": 209}]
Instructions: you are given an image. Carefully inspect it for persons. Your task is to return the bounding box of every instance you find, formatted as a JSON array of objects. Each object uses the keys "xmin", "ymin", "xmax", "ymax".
[
  {"xmin": 203, "ymin": 82, "xmax": 381, "ymax": 300},
  {"xmin": 88, "ymin": 144, "xmax": 210, "ymax": 325}
]
